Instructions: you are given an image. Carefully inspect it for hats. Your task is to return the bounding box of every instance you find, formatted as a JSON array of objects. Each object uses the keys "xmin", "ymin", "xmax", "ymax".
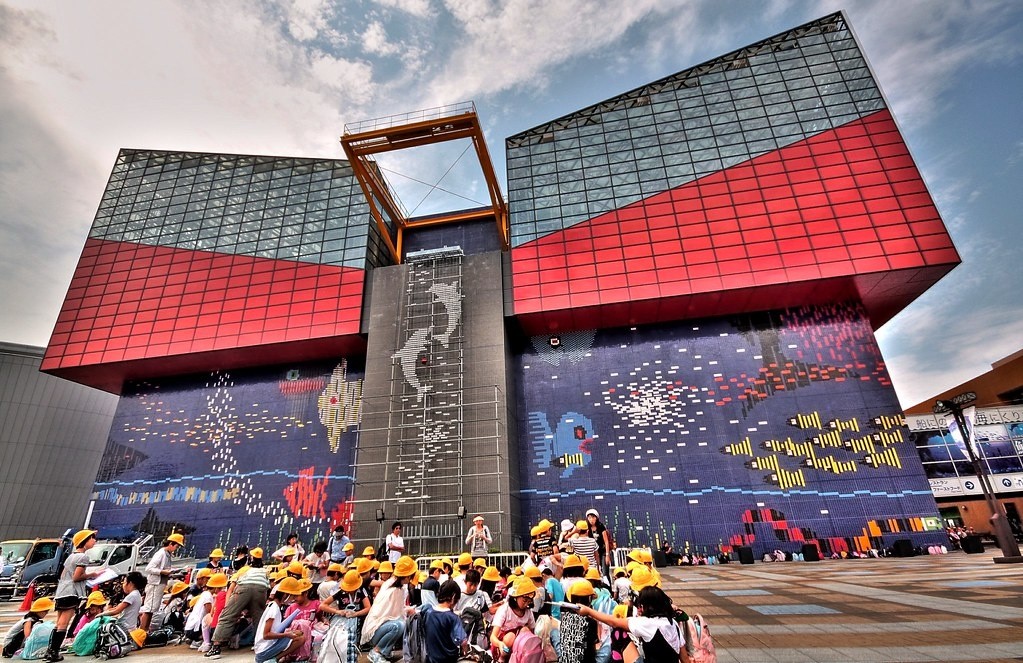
[
  {"xmin": 30, "ymin": 597, "xmax": 55, "ymax": 612},
  {"xmin": 73, "ymin": 529, "xmax": 98, "ymax": 548},
  {"xmin": 170, "ymin": 509, "xmax": 660, "ymax": 621},
  {"xmin": 167, "ymin": 533, "xmax": 184, "ymax": 547},
  {"xmin": 130, "ymin": 629, "xmax": 146, "ymax": 648},
  {"xmin": 85, "ymin": 591, "xmax": 107, "ymax": 609}
]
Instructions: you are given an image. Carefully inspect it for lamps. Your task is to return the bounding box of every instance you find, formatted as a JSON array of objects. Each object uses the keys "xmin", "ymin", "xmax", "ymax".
[
  {"xmin": 936, "ymin": 399, "xmax": 950, "ymax": 407},
  {"xmin": 457, "ymin": 506, "xmax": 467, "ymax": 519},
  {"xmin": 376, "ymin": 508, "xmax": 385, "ymax": 524}
]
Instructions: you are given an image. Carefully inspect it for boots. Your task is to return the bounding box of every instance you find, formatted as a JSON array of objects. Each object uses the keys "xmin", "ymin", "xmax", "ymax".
[
  {"xmin": 43, "ymin": 629, "xmax": 55, "ymax": 660},
  {"xmin": 47, "ymin": 629, "xmax": 66, "ymax": 663}
]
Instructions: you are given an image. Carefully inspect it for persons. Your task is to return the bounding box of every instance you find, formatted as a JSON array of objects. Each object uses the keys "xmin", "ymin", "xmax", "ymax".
[
  {"xmin": 662, "ymin": 542, "xmax": 675, "ymax": 564},
  {"xmin": 43, "ymin": 530, "xmax": 98, "ymax": 663},
  {"xmin": 272, "ymin": 533, "xmax": 305, "ymax": 563},
  {"xmin": 466, "ymin": 515, "xmax": 493, "ymax": 561},
  {"xmin": 139, "ymin": 534, "xmax": 184, "ymax": 631},
  {"xmin": 327, "ymin": 525, "xmax": 350, "ymax": 565},
  {"xmin": 386, "ymin": 522, "xmax": 404, "ymax": 568},
  {"xmin": 946, "ymin": 526, "xmax": 974, "ymax": 549},
  {"xmin": 2, "ymin": 597, "xmax": 54, "ymax": 658},
  {"xmin": 164, "ymin": 542, "xmax": 686, "ymax": 663},
  {"xmin": 73, "ymin": 591, "xmax": 107, "ymax": 637},
  {"xmin": 96, "ymin": 572, "xmax": 147, "ymax": 633},
  {"xmin": 529, "ymin": 509, "xmax": 611, "ymax": 575}
]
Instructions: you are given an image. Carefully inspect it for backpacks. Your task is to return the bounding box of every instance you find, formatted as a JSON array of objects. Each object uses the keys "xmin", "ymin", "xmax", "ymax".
[
  {"xmin": 72, "ymin": 615, "xmax": 117, "ymax": 657},
  {"xmin": 94, "ymin": 621, "xmax": 132, "ymax": 660},
  {"xmin": 401, "ymin": 606, "xmax": 598, "ymax": 663},
  {"xmin": 684, "ymin": 612, "xmax": 718, "ymax": 663},
  {"xmin": 279, "ymin": 604, "xmax": 358, "ymax": 663}
]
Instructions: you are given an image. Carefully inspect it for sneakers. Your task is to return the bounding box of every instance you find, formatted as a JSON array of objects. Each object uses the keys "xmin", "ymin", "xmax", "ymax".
[
  {"xmin": 203, "ymin": 644, "xmax": 221, "ymax": 660},
  {"xmin": 197, "ymin": 643, "xmax": 212, "ymax": 652},
  {"xmin": 366, "ymin": 649, "xmax": 391, "ymax": 663},
  {"xmin": 186, "ymin": 638, "xmax": 192, "ymax": 644},
  {"xmin": 190, "ymin": 641, "xmax": 204, "ymax": 649}
]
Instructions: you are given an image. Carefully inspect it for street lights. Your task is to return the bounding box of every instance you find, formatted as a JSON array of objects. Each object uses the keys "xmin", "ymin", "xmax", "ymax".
[{"xmin": 934, "ymin": 397, "xmax": 1023, "ymax": 564}]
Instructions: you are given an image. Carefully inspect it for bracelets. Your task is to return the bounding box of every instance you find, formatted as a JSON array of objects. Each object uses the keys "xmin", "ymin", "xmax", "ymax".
[
  {"xmin": 606, "ymin": 553, "xmax": 610, "ymax": 556},
  {"xmin": 102, "ymin": 612, "xmax": 106, "ymax": 616}
]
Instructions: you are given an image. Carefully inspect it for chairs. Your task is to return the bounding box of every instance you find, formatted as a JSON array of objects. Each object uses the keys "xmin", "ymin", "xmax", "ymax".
[{"xmin": 31, "ymin": 552, "xmax": 47, "ymax": 566}]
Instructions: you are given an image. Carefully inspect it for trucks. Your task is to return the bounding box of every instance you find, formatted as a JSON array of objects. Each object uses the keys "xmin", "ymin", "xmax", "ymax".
[
  {"xmin": 0, "ymin": 529, "xmax": 80, "ymax": 601},
  {"xmin": 82, "ymin": 542, "xmax": 198, "ymax": 595}
]
